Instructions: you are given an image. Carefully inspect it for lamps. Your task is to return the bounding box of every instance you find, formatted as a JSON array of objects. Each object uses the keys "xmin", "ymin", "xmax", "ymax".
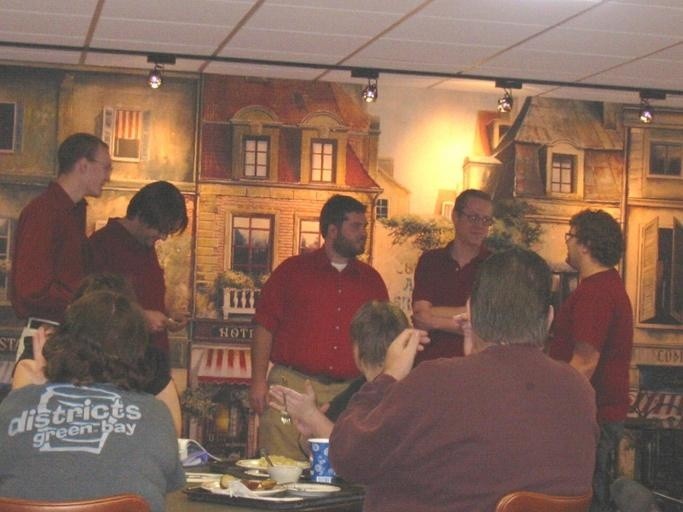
[{"xmin": 145, "ymin": 53, "xmax": 667, "ymax": 124}]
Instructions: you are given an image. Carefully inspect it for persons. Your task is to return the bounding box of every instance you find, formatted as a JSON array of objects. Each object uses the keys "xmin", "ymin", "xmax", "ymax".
[
  {"xmin": 267, "ymin": 300, "xmax": 411, "ymax": 439},
  {"xmin": 10, "ymin": 267, "xmax": 183, "ymax": 440},
  {"xmin": 10, "ymin": 132, "xmax": 112, "ymax": 318},
  {"xmin": 248, "ymin": 195, "xmax": 389, "ymax": 461},
  {"xmin": 325, "ymin": 247, "xmax": 601, "ymax": 510},
  {"xmin": 87, "ymin": 180, "xmax": 188, "ymax": 371},
  {"xmin": 411, "ymin": 187, "xmax": 496, "ymax": 365},
  {"xmin": 1, "ymin": 292, "xmax": 186, "ymax": 510},
  {"xmin": 551, "ymin": 208, "xmax": 633, "ymax": 503}
]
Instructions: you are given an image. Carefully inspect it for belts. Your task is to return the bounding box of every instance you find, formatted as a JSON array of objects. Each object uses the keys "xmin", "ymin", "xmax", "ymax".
[
  {"xmin": 279, "ymin": 361, "xmax": 353, "ymax": 384},
  {"xmin": 29, "ymin": 320, "xmax": 56, "ymax": 331}
]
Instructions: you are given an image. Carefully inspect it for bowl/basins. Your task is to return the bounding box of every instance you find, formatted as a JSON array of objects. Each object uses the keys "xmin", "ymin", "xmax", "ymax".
[{"xmin": 268, "ymin": 463, "xmax": 303, "ymax": 485}]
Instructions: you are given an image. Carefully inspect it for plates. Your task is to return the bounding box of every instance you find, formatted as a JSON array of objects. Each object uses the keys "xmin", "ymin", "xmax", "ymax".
[
  {"xmin": 202, "ymin": 480, "xmax": 287, "ymax": 495},
  {"xmin": 288, "ymin": 481, "xmax": 341, "ymax": 497},
  {"xmin": 245, "ymin": 471, "xmax": 306, "ymax": 481},
  {"xmin": 234, "ymin": 456, "xmax": 309, "ymax": 470}
]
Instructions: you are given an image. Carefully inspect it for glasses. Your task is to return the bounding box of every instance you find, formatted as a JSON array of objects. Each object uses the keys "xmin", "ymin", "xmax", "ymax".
[
  {"xmin": 565, "ymin": 233, "xmax": 577, "ymax": 241},
  {"xmin": 86, "ymin": 156, "xmax": 113, "ymax": 173},
  {"xmin": 460, "ymin": 211, "xmax": 493, "ymax": 225}
]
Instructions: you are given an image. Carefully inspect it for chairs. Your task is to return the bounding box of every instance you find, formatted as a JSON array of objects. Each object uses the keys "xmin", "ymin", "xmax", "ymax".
[
  {"xmin": 0, "ymin": 496, "xmax": 150, "ymax": 512},
  {"xmin": 494, "ymin": 491, "xmax": 597, "ymax": 511}
]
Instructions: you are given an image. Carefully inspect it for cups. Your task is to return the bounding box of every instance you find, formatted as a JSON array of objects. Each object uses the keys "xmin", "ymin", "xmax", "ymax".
[{"xmin": 308, "ymin": 439, "xmax": 336, "ymax": 483}]
